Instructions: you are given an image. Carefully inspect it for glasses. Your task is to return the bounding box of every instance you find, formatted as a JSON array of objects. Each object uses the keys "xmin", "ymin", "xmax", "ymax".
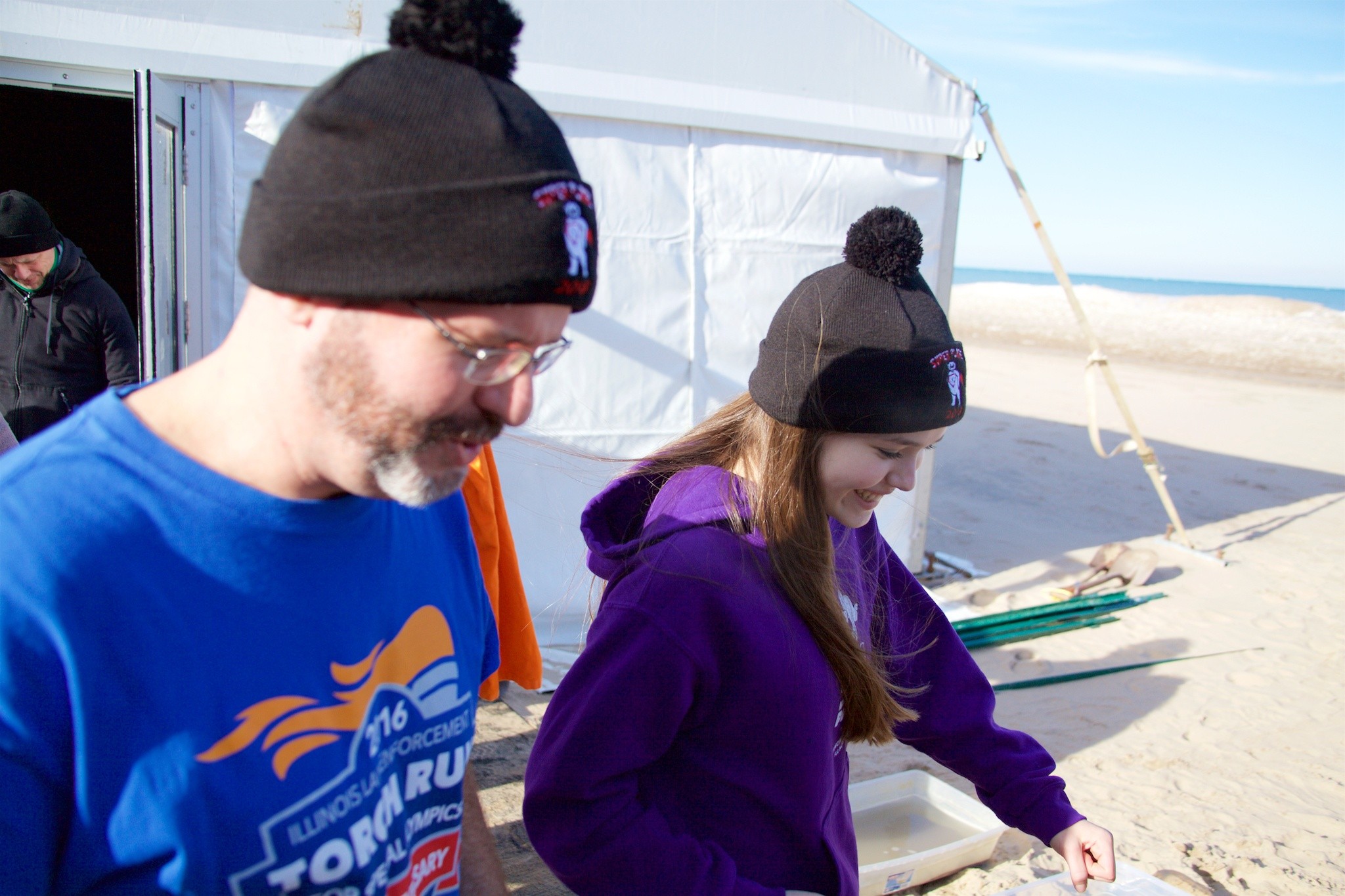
[{"xmin": 410, "ymin": 301, "xmax": 573, "ymax": 383}]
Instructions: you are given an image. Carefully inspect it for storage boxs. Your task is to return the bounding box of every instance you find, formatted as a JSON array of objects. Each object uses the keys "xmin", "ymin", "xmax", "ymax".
[{"xmin": 848, "ymin": 767, "xmax": 1012, "ymax": 896}]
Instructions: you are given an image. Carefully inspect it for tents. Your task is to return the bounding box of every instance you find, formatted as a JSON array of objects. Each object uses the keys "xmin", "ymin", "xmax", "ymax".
[{"xmin": 0, "ymin": 0, "xmax": 976, "ymax": 651}]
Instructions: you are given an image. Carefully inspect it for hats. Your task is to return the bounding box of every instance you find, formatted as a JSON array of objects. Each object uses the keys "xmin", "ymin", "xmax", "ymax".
[
  {"xmin": 237, "ymin": 1, "xmax": 597, "ymax": 310},
  {"xmin": 1, "ymin": 191, "xmax": 63, "ymax": 259},
  {"xmin": 747, "ymin": 210, "xmax": 968, "ymax": 434}
]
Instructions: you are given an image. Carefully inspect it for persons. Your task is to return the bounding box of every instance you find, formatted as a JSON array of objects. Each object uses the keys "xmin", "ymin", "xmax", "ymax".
[
  {"xmin": 0, "ymin": 189, "xmax": 139, "ymax": 444},
  {"xmin": 0, "ymin": 0, "xmax": 597, "ymax": 896},
  {"xmin": 521, "ymin": 207, "xmax": 1116, "ymax": 896}
]
